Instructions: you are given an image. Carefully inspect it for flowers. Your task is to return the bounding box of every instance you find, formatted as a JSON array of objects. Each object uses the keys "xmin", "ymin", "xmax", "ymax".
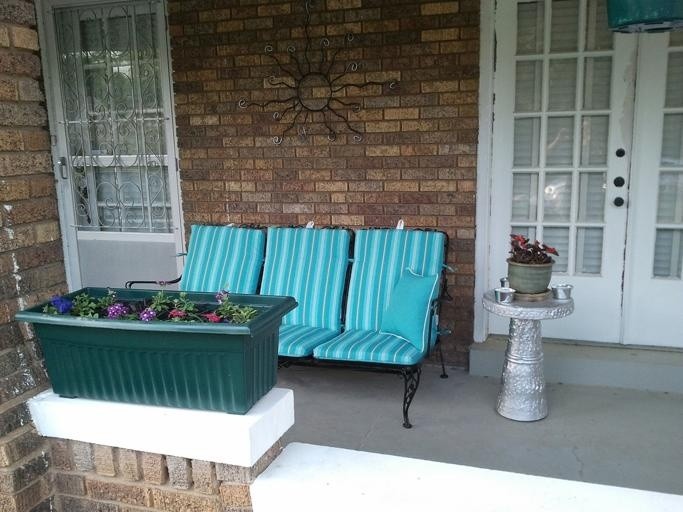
[
  {"xmin": 509, "ymin": 234, "xmax": 559, "ymax": 264},
  {"xmin": 43, "ymin": 287, "xmax": 261, "ymax": 324}
]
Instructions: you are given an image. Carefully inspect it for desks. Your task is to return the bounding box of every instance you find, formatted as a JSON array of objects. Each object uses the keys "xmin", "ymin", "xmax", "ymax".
[{"xmin": 482, "ymin": 287, "xmax": 574, "ymax": 422}]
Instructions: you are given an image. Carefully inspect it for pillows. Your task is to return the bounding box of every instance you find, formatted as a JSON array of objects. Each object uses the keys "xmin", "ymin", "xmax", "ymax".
[{"xmin": 378, "ymin": 267, "xmax": 438, "ymax": 353}]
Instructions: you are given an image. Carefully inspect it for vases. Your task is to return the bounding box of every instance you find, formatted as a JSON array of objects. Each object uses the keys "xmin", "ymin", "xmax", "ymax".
[{"xmin": 507, "ymin": 257, "xmax": 555, "ymax": 294}]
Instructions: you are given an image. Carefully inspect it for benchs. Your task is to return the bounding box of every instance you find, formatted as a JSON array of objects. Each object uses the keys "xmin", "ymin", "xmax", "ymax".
[{"xmin": 125, "ymin": 222, "xmax": 454, "ymax": 429}]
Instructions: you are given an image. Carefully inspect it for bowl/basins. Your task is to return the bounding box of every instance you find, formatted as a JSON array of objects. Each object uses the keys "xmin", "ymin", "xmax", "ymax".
[
  {"xmin": 494, "ymin": 287, "xmax": 516, "ymax": 306},
  {"xmin": 499, "ymin": 277, "xmax": 510, "ymax": 287},
  {"xmin": 551, "ymin": 283, "xmax": 573, "ymax": 300}
]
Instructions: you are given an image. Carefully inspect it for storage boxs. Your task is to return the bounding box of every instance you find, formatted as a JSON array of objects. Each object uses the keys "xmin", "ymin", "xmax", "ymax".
[{"xmin": 14, "ymin": 287, "xmax": 298, "ymax": 415}]
